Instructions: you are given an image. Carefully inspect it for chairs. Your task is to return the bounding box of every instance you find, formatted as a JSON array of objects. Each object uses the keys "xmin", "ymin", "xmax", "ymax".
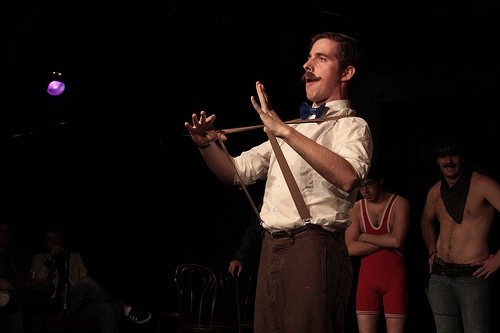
[{"xmin": 165, "ymin": 260, "xmax": 253, "ymax": 333}]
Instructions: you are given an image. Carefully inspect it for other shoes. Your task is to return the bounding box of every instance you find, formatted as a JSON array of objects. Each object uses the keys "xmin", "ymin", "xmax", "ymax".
[{"xmin": 126, "ymin": 306, "xmax": 151, "ymax": 324}]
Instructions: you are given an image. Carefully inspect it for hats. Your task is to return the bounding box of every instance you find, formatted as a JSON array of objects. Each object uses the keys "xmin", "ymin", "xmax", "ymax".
[{"xmin": 425, "ymin": 133, "xmax": 466, "ymax": 163}]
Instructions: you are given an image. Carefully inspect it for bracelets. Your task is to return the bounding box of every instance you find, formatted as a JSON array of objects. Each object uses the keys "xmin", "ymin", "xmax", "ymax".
[
  {"xmin": 43, "ymin": 259, "xmax": 53, "ymax": 268},
  {"xmin": 198, "ymin": 144, "xmax": 211, "ymax": 149},
  {"xmin": 430, "ymin": 251, "xmax": 437, "ymax": 257}
]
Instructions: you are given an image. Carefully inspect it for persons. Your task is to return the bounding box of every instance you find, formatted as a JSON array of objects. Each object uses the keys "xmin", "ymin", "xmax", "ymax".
[
  {"xmin": 185, "ymin": 32, "xmax": 372, "ymax": 333},
  {"xmin": 229, "ymin": 216, "xmax": 263, "ymax": 283},
  {"xmin": 422, "ymin": 141, "xmax": 500, "ymax": 333},
  {"xmin": 0, "ymin": 213, "xmax": 24, "ymax": 333},
  {"xmin": 345, "ymin": 170, "xmax": 410, "ymax": 333},
  {"xmin": 32, "ymin": 224, "xmax": 152, "ymax": 333}
]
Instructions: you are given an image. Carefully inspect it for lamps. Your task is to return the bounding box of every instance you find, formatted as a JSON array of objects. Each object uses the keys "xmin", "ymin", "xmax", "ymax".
[{"xmin": 45, "ymin": 63, "xmax": 67, "ymax": 96}]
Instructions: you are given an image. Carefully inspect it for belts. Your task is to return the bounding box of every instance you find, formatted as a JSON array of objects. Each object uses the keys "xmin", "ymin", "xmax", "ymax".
[{"xmin": 431, "ymin": 262, "xmax": 483, "ymax": 278}]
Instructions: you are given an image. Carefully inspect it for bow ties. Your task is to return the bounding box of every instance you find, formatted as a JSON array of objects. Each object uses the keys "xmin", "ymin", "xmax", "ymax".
[{"xmin": 298, "ymin": 102, "xmax": 326, "ymax": 119}]
